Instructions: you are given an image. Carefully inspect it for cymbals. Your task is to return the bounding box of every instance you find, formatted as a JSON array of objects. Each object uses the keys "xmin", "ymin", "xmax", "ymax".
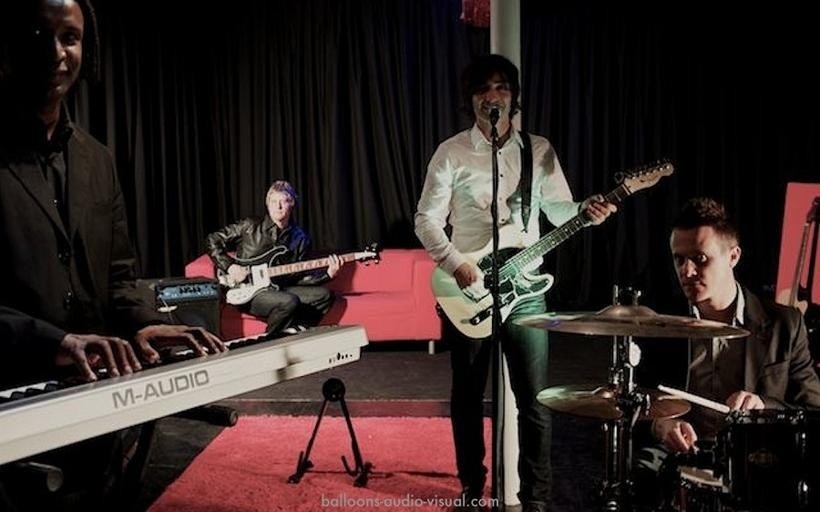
[
  {"xmin": 512, "ymin": 304, "xmax": 750, "ymax": 340},
  {"xmin": 537, "ymin": 385, "xmax": 691, "ymax": 421}
]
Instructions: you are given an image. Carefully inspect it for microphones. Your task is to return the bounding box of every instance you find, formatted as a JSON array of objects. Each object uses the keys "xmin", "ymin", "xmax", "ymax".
[{"xmin": 487, "ymin": 105, "xmax": 502, "ymax": 126}]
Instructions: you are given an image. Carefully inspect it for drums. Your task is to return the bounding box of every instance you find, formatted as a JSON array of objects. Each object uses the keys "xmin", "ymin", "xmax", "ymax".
[
  {"xmin": 716, "ymin": 405, "xmax": 820, "ymax": 511},
  {"xmin": 673, "ymin": 463, "xmax": 720, "ymax": 512}
]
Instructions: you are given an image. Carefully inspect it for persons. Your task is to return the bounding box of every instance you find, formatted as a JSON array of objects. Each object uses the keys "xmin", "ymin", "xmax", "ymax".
[
  {"xmin": 205, "ymin": 179, "xmax": 345, "ymax": 334},
  {"xmin": 636, "ymin": 195, "xmax": 820, "ymax": 455},
  {"xmin": 412, "ymin": 53, "xmax": 621, "ymax": 512},
  {"xmin": 2, "ymin": 0, "xmax": 228, "ymax": 381}
]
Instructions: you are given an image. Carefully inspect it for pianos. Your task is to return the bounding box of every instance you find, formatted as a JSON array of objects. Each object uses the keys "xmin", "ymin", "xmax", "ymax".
[{"xmin": 0, "ymin": 324, "xmax": 371, "ymax": 467}]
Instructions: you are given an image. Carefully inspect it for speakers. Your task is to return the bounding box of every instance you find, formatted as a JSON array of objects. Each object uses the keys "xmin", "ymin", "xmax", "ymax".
[{"xmin": 135, "ymin": 275, "xmax": 222, "ymax": 340}]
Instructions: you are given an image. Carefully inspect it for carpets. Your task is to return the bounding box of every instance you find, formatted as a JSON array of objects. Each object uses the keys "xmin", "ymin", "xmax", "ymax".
[{"xmin": 149, "ymin": 414, "xmax": 492, "ymax": 512}]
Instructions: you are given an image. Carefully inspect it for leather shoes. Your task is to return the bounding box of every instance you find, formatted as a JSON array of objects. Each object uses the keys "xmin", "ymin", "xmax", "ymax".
[{"xmin": 459, "ymin": 488, "xmax": 484, "ymax": 512}]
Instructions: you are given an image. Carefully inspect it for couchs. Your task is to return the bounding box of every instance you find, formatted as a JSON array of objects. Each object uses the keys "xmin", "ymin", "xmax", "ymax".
[{"xmin": 185, "ymin": 248, "xmax": 444, "ymax": 357}]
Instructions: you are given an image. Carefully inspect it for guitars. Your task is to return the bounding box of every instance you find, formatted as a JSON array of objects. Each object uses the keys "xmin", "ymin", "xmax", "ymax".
[
  {"xmin": 212, "ymin": 241, "xmax": 380, "ymax": 307},
  {"xmin": 429, "ymin": 157, "xmax": 675, "ymax": 343}
]
